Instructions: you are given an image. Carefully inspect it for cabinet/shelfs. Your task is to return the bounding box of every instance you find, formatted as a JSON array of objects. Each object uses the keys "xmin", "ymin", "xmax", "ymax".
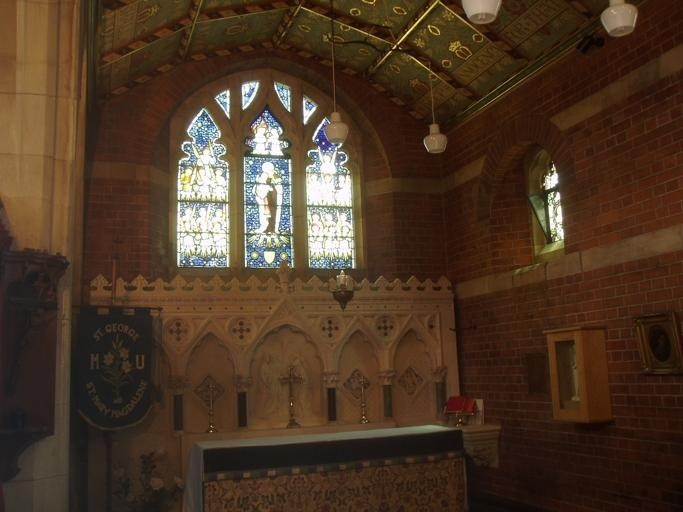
[
  {"xmin": 542, "ymin": 323, "xmax": 613, "ymax": 425},
  {"xmin": 0, "ymin": 249, "xmax": 73, "ymax": 479}
]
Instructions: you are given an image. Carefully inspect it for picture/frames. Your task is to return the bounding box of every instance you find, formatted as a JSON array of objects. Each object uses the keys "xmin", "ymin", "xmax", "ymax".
[{"xmin": 632, "ymin": 310, "xmax": 683, "ymax": 377}]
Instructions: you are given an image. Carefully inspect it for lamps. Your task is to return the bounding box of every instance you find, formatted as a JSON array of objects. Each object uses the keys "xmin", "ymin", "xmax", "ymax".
[
  {"xmin": 328, "ymin": 270, "xmax": 358, "ymax": 314},
  {"xmin": 600, "ymin": 0, "xmax": 639, "ymax": 40},
  {"xmin": 323, "ymin": 4, "xmax": 351, "ymax": 144},
  {"xmin": 421, "ymin": 40, "xmax": 448, "ymax": 155},
  {"xmin": 459, "ymin": 0, "xmax": 499, "ymax": 26}
]
